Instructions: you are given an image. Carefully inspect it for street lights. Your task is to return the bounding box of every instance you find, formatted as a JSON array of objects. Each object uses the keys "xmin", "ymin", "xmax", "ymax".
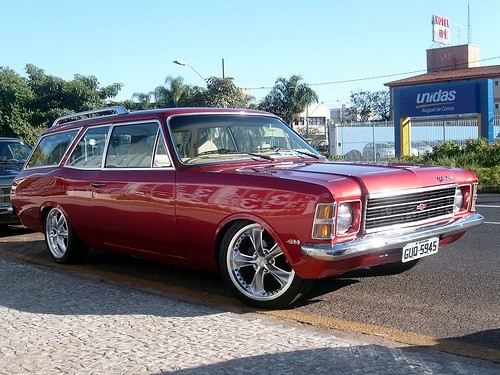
[{"xmin": 173, "ymin": 59, "xmax": 207, "ymax": 83}]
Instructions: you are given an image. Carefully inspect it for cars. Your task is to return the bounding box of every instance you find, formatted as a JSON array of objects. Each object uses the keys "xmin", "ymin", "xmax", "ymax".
[
  {"xmin": 0, "ymin": 137, "xmax": 45, "ymax": 228},
  {"xmin": 9, "ymin": 104, "xmax": 486, "ymax": 311}
]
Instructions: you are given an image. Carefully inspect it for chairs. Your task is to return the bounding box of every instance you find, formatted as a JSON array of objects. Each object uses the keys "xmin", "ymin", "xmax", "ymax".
[{"xmin": 194, "ymin": 138, "xmax": 218, "ymax": 155}]
